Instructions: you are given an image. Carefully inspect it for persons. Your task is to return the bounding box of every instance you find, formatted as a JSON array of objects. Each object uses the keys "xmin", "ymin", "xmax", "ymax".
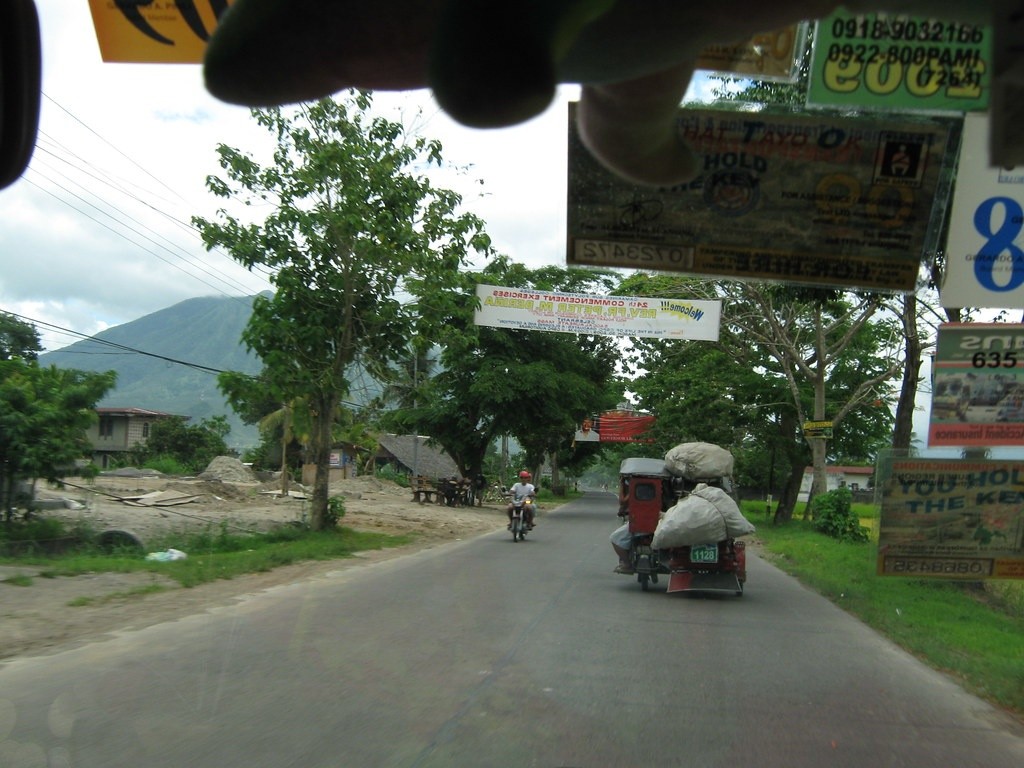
[
  {"xmin": 506, "ymin": 470, "xmax": 538, "ymax": 530},
  {"xmin": 611, "ymin": 475, "xmax": 652, "ymax": 574},
  {"xmin": 203, "ymin": 0, "xmax": 883, "ymax": 189},
  {"xmin": 574, "ymin": 416, "xmax": 600, "ymax": 441}
]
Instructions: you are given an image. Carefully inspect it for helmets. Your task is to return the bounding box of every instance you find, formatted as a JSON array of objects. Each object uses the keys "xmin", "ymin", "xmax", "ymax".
[{"xmin": 520, "ymin": 471, "xmax": 528, "ymax": 477}]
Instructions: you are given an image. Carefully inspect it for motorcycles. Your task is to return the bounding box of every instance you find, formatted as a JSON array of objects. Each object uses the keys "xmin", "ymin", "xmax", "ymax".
[
  {"xmin": 499, "ymin": 485, "xmax": 539, "ymax": 543},
  {"xmin": 617, "ymin": 457, "xmax": 747, "ymax": 596}
]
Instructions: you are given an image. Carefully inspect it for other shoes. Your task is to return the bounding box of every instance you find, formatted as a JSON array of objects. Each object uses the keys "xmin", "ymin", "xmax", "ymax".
[
  {"xmin": 616, "ymin": 559, "xmax": 630, "ymax": 571},
  {"xmin": 507, "ymin": 526, "xmax": 510, "ymax": 529},
  {"xmin": 507, "ymin": 523, "xmax": 511, "ymax": 526},
  {"xmin": 531, "ymin": 522, "xmax": 536, "ymax": 526},
  {"xmin": 528, "ymin": 525, "xmax": 532, "ymax": 531}
]
